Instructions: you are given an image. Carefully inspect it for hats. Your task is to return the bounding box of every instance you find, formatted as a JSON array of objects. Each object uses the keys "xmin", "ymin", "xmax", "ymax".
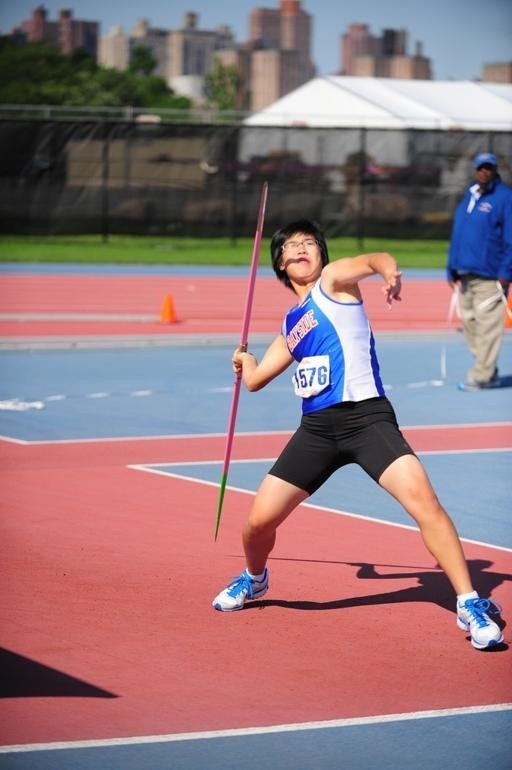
[{"xmin": 473, "ymin": 152, "xmax": 497, "ymax": 168}]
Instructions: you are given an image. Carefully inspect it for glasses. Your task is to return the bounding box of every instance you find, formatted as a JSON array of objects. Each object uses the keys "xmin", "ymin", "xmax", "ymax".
[{"xmin": 279, "ymin": 238, "xmax": 321, "ymax": 250}]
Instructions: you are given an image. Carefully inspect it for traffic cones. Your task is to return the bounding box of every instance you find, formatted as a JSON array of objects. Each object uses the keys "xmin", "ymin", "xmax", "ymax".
[
  {"xmin": 503, "ymin": 293, "xmax": 512, "ymax": 330},
  {"xmin": 159, "ymin": 289, "xmax": 176, "ymax": 323}
]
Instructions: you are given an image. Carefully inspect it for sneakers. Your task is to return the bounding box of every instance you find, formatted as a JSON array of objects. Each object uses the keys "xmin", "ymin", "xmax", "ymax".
[
  {"xmin": 456, "ymin": 594, "xmax": 505, "ymax": 650},
  {"xmin": 211, "ymin": 568, "xmax": 269, "ymax": 612},
  {"xmin": 458, "ymin": 369, "xmax": 502, "ymax": 391}
]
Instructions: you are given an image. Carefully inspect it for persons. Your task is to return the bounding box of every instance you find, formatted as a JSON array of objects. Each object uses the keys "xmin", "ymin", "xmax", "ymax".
[
  {"xmin": 446, "ymin": 151, "xmax": 512, "ymax": 393},
  {"xmin": 210, "ymin": 218, "xmax": 505, "ymax": 651}
]
